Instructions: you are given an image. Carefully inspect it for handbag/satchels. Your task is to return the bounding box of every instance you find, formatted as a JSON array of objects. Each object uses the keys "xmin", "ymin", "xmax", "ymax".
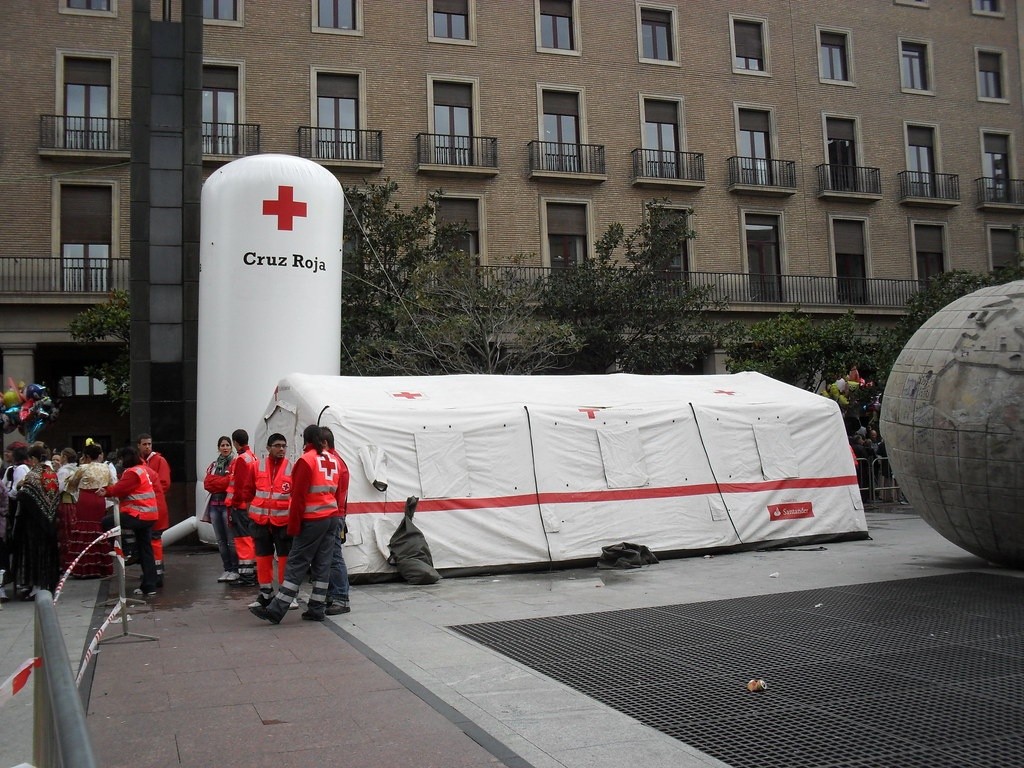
[{"xmin": 341, "ymin": 521, "xmax": 348, "ymax": 544}]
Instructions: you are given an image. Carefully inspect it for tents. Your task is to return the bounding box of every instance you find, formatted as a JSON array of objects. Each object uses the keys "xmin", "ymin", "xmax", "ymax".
[{"xmin": 262, "ymin": 366, "xmax": 874, "ymax": 579}]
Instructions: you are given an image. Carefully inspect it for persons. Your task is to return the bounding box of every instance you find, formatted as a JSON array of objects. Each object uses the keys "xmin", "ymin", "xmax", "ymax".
[
  {"xmin": 0, "ymin": 432, "xmax": 172, "ymax": 602},
  {"xmin": 249, "ymin": 425, "xmax": 352, "ymax": 624},
  {"xmin": 850, "ymin": 426, "xmax": 910, "ymax": 512},
  {"xmin": 225, "ymin": 429, "xmax": 261, "ymax": 587},
  {"xmin": 244, "ymin": 433, "xmax": 301, "ymax": 610},
  {"xmin": 203, "ymin": 436, "xmax": 242, "ymax": 581}
]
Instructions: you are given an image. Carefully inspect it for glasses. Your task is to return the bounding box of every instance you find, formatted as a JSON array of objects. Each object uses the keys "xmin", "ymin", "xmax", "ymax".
[{"xmin": 271, "ymin": 445, "xmax": 288, "ymax": 448}]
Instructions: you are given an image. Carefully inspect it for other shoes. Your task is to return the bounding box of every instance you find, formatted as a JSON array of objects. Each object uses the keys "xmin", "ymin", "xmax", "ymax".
[
  {"xmin": 248, "ymin": 601, "xmax": 262, "ymax": 608},
  {"xmin": 140, "ymin": 574, "xmax": 162, "ymax": 587},
  {"xmin": 218, "ymin": 571, "xmax": 240, "ymax": 582},
  {"xmin": 325, "ymin": 602, "xmax": 351, "ymax": 615},
  {"xmin": 134, "ymin": 588, "xmax": 156, "ymax": 595},
  {"xmin": 290, "ymin": 598, "xmax": 299, "ymax": 609},
  {"xmin": 16, "ymin": 583, "xmax": 40, "ymax": 601},
  {"xmin": 238, "ymin": 574, "xmax": 256, "ymax": 586},
  {"xmin": 302, "ymin": 606, "xmax": 325, "ymax": 621},
  {"xmin": 250, "ymin": 607, "xmax": 283, "ymax": 624}
]
